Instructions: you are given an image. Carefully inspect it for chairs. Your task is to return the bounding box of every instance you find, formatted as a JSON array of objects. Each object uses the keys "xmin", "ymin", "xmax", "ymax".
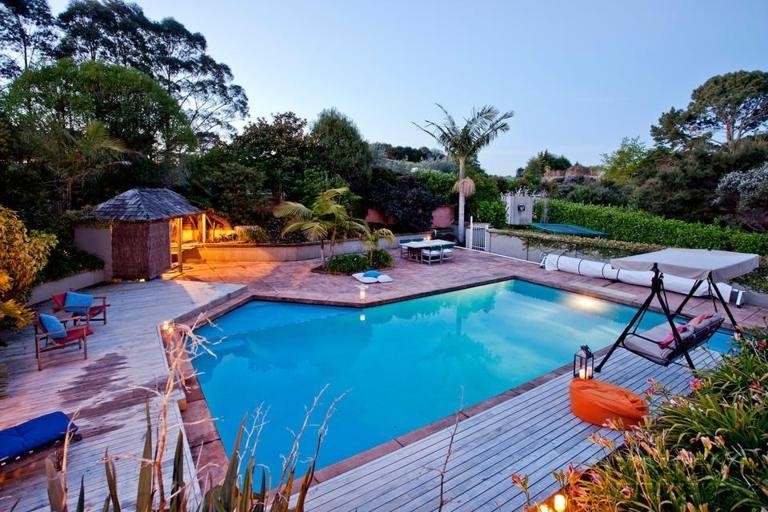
[
  {"xmin": 30, "ymin": 289, "xmax": 107, "ymax": 371},
  {"xmin": 399, "ymin": 238, "xmax": 456, "ymax": 265}
]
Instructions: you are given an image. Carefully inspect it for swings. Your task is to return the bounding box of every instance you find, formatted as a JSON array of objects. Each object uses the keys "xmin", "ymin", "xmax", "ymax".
[{"xmin": 617, "ymin": 277, "xmax": 724, "ymax": 367}]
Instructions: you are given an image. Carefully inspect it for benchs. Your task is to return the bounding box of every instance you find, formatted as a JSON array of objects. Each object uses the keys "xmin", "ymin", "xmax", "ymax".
[
  {"xmin": 0, "ymin": 411, "xmax": 82, "ymax": 464},
  {"xmin": 569, "ymin": 377, "xmax": 649, "ymax": 433}
]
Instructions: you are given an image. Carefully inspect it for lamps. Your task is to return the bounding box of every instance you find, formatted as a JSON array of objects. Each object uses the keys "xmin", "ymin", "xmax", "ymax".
[{"xmin": 573, "ymin": 346, "xmax": 595, "ymax": 380}]
